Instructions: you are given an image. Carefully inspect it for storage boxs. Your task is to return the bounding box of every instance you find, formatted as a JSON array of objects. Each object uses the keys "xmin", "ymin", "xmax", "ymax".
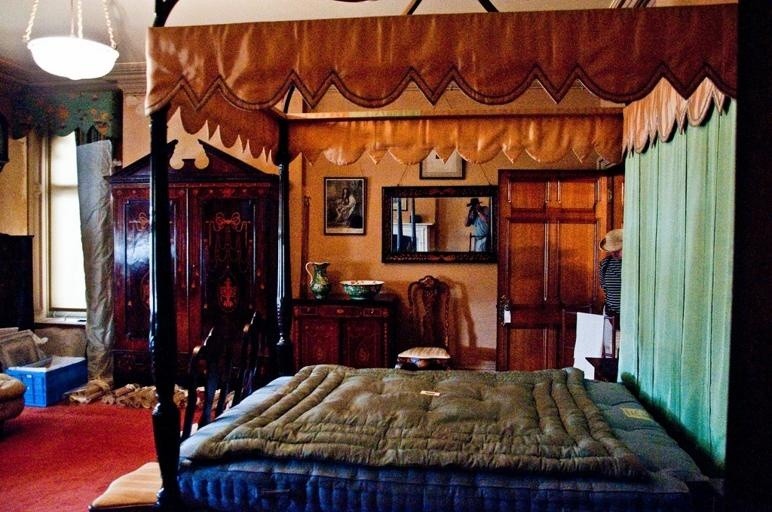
[{"xmin": 6, "ymin": 354, "xmax": 88, "ymax": 408}]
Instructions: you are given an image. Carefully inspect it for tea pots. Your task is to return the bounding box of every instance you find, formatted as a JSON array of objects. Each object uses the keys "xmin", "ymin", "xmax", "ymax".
[{"xmin": 304, "ymin": 262, "xmax": 331, "ymax": 301}]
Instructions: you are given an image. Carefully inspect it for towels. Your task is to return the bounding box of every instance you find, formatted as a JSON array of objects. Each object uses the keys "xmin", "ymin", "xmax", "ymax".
[{"xmin": 573, "ymin": 311, "xmax": 612, "ymax": 379}]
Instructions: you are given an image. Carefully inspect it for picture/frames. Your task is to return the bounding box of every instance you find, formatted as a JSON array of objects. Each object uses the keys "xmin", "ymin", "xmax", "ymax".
[
  {"xmin": 419, "ymin": 147, "xmax": 467, "ymax": 180},
  {"xmin": 393, "ymin": 198, "xmax": 408, "ymax": 211},
  {"xmin": 323, "ymin": 175, "xmax": 368, "ymax": 237}
]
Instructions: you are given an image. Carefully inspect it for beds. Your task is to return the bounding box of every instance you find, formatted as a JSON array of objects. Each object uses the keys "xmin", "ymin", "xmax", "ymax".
[{"xmin": 143, "ymin": 0, "xmax": 769, "ymax": 508}]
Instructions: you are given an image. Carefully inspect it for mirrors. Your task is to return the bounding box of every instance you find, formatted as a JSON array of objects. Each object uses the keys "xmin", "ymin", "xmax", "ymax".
[{"xmin": 381, "ymin": 184, "xmax": 497, "ymax": 263}]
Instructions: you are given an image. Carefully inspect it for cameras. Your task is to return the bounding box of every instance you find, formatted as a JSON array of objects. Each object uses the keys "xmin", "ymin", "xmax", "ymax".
[{"xmin": 471, "ymin": 203, "xmax": 477, "ymax": 211}]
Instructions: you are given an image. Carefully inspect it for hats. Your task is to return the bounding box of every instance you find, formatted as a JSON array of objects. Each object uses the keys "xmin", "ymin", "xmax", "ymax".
[
  {"xmin": 465, "ymin": 198, "xmax": 483, "ymax": 207},
  {"xmin": 598, "ymin": 229, "xmax": 623, "ymax": 253}
]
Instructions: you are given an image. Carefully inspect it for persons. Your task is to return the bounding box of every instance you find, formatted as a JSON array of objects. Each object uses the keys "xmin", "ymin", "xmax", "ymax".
[
  {"xmin": 596, "ymin": 229, "xmax": 622, "ymax": 359},
  {"xmin": 462, "ymin": 199, "xmax": 489, "ymax": 252}
]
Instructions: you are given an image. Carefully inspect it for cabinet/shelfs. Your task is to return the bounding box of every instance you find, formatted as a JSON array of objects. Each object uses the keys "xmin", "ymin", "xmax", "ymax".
[
  {"xmin": 103, "ymin": 137, "xmax": 277, "ymax": 389},
  {"xmin": 290, "ymin": 296, "xmax": 401, "ymax": 369}
]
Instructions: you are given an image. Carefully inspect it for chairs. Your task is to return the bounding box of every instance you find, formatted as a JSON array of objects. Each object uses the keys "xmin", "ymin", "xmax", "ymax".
[
  {"xmin": 87, "ymin": 328, "xmax": 231, "ymax": 512},
  {"xmin": 180, "ymin": 311, "xmax": 268, "ymax": 442},
  {"xmin": 395, "ymin": 274, "xmax": 454, "ymax": 371}
]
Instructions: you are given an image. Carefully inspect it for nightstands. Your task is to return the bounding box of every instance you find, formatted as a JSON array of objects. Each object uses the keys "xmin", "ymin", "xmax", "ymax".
[{"xmin": 584, "ymin": 355, "xmax": 618, "ymax": 382}]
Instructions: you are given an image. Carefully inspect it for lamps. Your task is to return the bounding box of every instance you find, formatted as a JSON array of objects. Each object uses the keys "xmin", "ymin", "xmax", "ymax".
[{"xmin": 24, "ymin": 0, "xmax": 122, "ymax": 80}]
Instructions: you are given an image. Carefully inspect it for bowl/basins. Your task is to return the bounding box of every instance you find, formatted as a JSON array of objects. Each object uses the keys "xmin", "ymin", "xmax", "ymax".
[{"xmin": 339, "ymin": 279, "xmax": 384, "ymax": 302}]
